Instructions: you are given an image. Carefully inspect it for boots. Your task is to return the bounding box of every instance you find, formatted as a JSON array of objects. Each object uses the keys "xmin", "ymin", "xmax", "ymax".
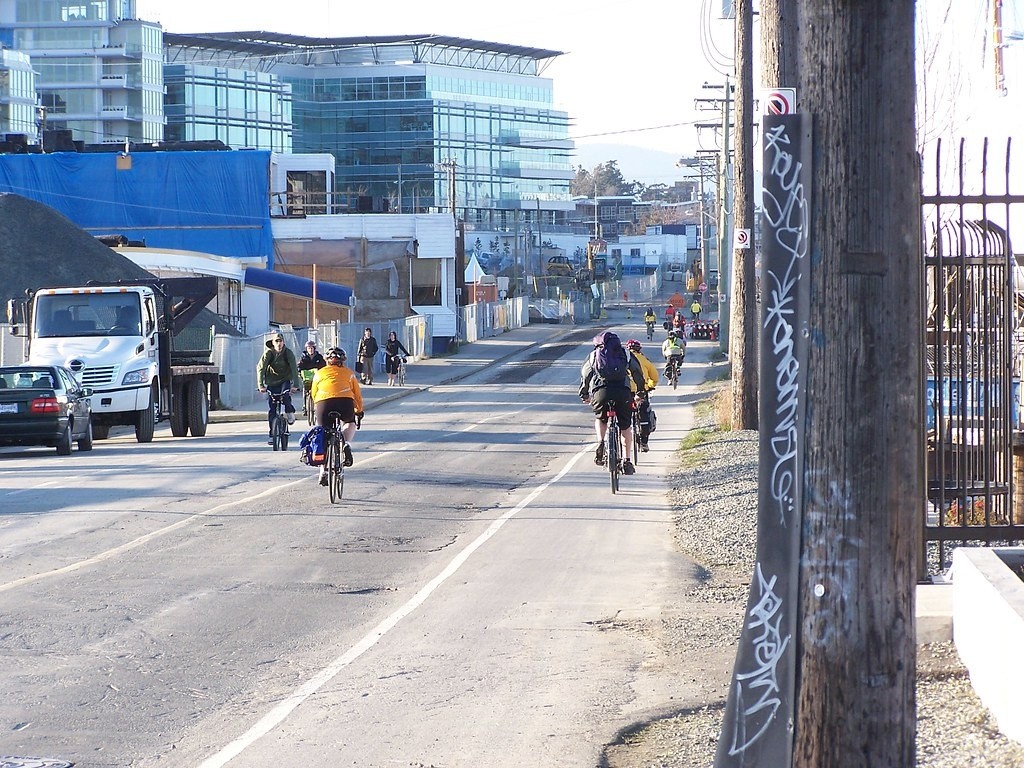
[{"xmin": 387, "ymin": 378, "xmax": 395, "ymax": 386}]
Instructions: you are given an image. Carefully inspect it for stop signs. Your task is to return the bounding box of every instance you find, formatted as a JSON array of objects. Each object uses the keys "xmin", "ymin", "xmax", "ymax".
[{"xmin": 699, "ymin": 283, "xmax": 707, "ymax": 292}]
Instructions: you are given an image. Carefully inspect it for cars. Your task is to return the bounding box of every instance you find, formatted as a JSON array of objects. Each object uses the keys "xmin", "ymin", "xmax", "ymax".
[{"xmin": 0, "ymin": 366, "xmax": 95, "ymax": 456}]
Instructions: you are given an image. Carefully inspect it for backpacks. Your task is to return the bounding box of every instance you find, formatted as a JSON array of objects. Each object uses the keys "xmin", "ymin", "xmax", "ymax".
[
  {"xmin": 646, "ymin": 307, "xmax": 653, "ymax": 316},
  {"xmin": 667, "ymin": 336, "xmax": 680, "ymax": 350},
  {"xmin": 594, "ymin": 332, "xmax": 627, "ymax": 382}
]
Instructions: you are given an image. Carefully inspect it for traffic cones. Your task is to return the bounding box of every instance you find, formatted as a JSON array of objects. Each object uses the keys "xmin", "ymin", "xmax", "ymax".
[
  {"xmin": 710, "ymin": 331, "xmax": 716, "ymax": 340},
  {"xmin": 626, "ymin": 308, "xmax": 632, "ymax": 319},
  {"xmin": 602, "ymin": 309, "xmax": 607, "ymax": 317}
]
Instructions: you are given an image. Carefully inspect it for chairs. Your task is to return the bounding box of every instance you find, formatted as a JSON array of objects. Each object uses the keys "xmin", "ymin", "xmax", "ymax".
[
  {"xmin": 33, "ymin": 380, "xmax": 51, "ymax": 387},
  {"xmin": 0, "ymin": 378, "xmax": 7, "ymax": 388},
  {"xmin": 112, "ymin": 305, "xmax": 140, "ymax": 334},
  {"xmin": 45, "ymin": 310, "xmax": 72, "ymax": 334}
]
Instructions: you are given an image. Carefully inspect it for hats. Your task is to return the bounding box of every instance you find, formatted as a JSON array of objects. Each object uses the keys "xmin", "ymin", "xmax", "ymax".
[{"xmin": 273, "ymin": 333, "xmax": 285, "ymax": 342}]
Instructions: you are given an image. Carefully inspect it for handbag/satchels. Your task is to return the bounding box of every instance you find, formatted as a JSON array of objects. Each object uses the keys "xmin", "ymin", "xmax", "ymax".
[
  {"xmin": 355, "ymin": 356, "xmax": 365, "ymax": 373},
  {"xmin": 299, "ymin": 426, "xmax": 329, "ymax": 466}
]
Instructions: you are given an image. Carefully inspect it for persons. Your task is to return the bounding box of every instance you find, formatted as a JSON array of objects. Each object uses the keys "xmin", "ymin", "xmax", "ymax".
[
  {"xmin": 357, "ymin": 328, "xmax": 378, "ymax": 385},
  {"xmin": 385, "ymin": 331, "xmax": 410, "ymax": 386},
  {"xmin": 297, "ymin": 341, "xmax": 326, "ymax": 416},
  {"xmin": 689, "ymin": 300, "xmax": 702, "ymax": 321},
  {"xmin": 643, "ymin": 307, "xmax": 657, "ymax": 339},
  {"xmin": 673, "ymin": 311, "xmax": 686, "ymax": 332},
  {"xmin": 665, "ymin": 305, "xmax": 675, "ymax": 326},
  {"xmin": 622, "ymin": 339, "xmax": 659, "ymax": 452},
  {"xmin": 661, "ymin": 331, "xmax": 686, "ymax": 386},
  {"xmin": 257, "ymin": 333, "xmax": 299, "ymax": 444},
  {"xmin": 311, "ymin": 347, "xmax": 363, "ymax": 486},
  {"xmin": 578, "ymin": 332, "xmax": 646, "ymax": 475}
]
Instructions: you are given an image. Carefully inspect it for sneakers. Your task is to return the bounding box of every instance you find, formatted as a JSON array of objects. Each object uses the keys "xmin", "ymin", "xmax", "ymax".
[
  {"xmin": 594, "ymin": 445, "xmax": 605, "ymax": 467},
  {"xmin": 268, "ymin": 436, "xmax": 274, "ymax": 445},
  {"xmin": 318, "ymin": 468, "xmax": 329, "ymax": 486},
  {"xmin": 641, "ymin": 443, "xmax": 650, "ymax": 452},
  {"xmin": 286, "ymin": 412, "xmax": 295, "ymax": 425},
  {"xmin": 342, "ymin": 444, "xmax": 353, "ymax": 467},
  {"xmin": 623, "ymin": 461, "xmax": 635, "ymax": 475}
]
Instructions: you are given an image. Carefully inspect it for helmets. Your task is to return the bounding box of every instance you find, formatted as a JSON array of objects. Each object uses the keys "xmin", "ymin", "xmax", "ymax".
[
  {"xmin": 323, "ymin": 346, "xmax": 347, "ymax": 363},
  {"xmin": 305, "ymin": 341, "xmax": 316, "ymax": 348},
  {"xmin": 625, "ymin": 338, "xmax": 642, "ymax": 350},
  {"xmin": 668, "ymin": 304, "xmax": 673, "ymax": 307},
  {"xmin": 693, "ymin": 300, "xmax": 697, "ymax": 303},
  {"xmin": 668, "ymin": 330, "xmax": 677, "ymax": 335}
]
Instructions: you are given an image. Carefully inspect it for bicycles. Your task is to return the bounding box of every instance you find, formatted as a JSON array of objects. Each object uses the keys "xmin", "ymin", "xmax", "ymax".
[
  {"xmin": 602, "ymin": 400, "xmax": 623, "ymax": 494},
  {"xmin": 302, "ymin": 380, "xmax": 317, "ymax": 426},
  {"xmin": 394, "ymin": 354, "xmax": 407, "ymax": 387},
  {"xmin": 649, "ymin": 321, "xmax": 653, "ymax": 341},
  {"xmin": 631, "ymin": 398, "xmax": 643, "ymax": 465},
  {"xmin": 665, "ymin": 312, "xmax": 718, "ymax": 339},
  {"xmin": 321, "ymin": 410, "xmax": 365, "ymax": 504},
  {"xmin": 253, "ymin": 387, "xmax": 300, "ymax": 450},
  {"xmin": 671, "ymin": 356, "xmax": 678, "ymax": 390}
]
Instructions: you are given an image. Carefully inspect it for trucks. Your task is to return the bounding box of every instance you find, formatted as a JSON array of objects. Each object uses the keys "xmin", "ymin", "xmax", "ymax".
[{"xmin": 7, "ymin": 277, "xmax": 226, "ymax": 442}]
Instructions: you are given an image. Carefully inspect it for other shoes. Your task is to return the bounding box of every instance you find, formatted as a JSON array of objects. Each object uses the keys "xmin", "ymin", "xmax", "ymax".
[
  {"xmin": 360, "ymin": 379, "xmax": 366, "ymax": 384},
  {"xmin": 667, "ymin": 380, "xmax": 673, "ymax": 385},
  {"xmin": 368, "ymin": 382, "xmax": 372, "ymax": 385},
  {"xmin": 677, "ymin": 370, "xmax": 682, "ymax": 375}
]
